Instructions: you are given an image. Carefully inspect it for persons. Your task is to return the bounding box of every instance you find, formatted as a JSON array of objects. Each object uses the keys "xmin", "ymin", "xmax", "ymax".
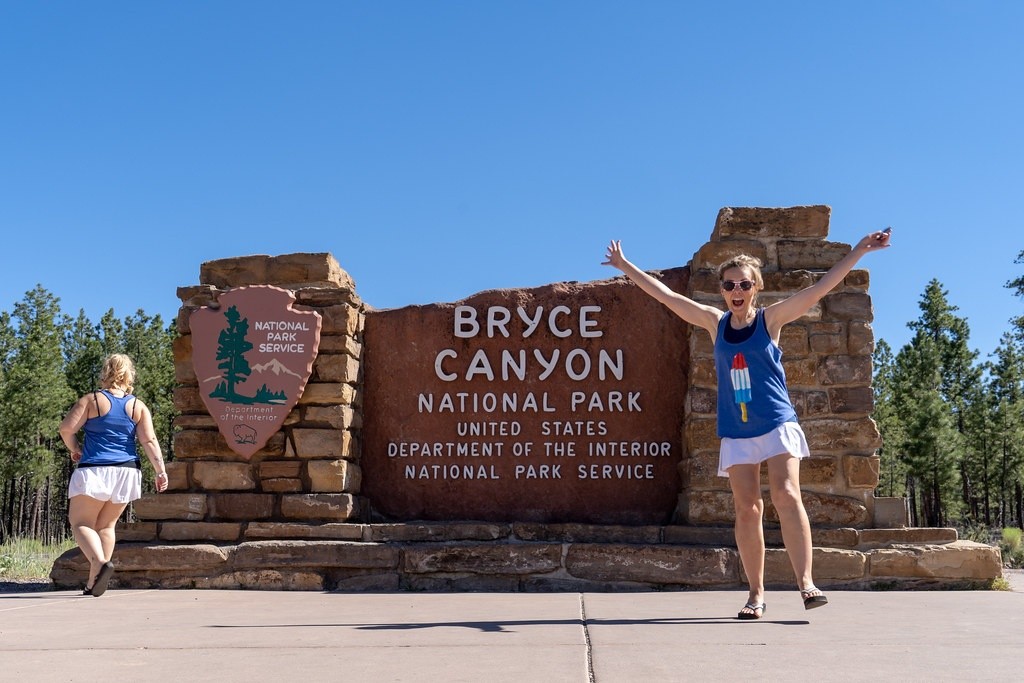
[
  {"xmin": 59, "ymin": 352, "xmax": 169, "ymax": 597},
  {"xmin": 599, "ymin": 226, "xmax": 892, "ymax": 621}
]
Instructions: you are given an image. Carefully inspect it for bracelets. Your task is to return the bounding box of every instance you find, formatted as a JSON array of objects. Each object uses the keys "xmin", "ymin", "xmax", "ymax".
[{"xmin": 156, "ymin": 472, "xmax": 167, "ymax": 477}]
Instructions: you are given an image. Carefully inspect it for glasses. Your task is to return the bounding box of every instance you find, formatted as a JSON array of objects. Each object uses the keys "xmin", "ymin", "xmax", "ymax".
[{"xmin": 723, "ymin": 281, "xmax": 755, "ymax": 292}]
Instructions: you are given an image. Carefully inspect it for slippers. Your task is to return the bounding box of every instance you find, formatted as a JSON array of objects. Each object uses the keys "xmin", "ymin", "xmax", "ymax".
[
  {"xmin": 801, "ymin": 588, "xmax": 828, "ymax": 611},
  {"xmin": 92, "ymin": 562, "xmax": 114, "ymax": 597},
  {"xmin": 738, "ymin": 603, "xmax": 766, "ymax": 620},
  {"xmin": 83, "ymin": 586, "xmax": 94, "ymax": 596}
]
